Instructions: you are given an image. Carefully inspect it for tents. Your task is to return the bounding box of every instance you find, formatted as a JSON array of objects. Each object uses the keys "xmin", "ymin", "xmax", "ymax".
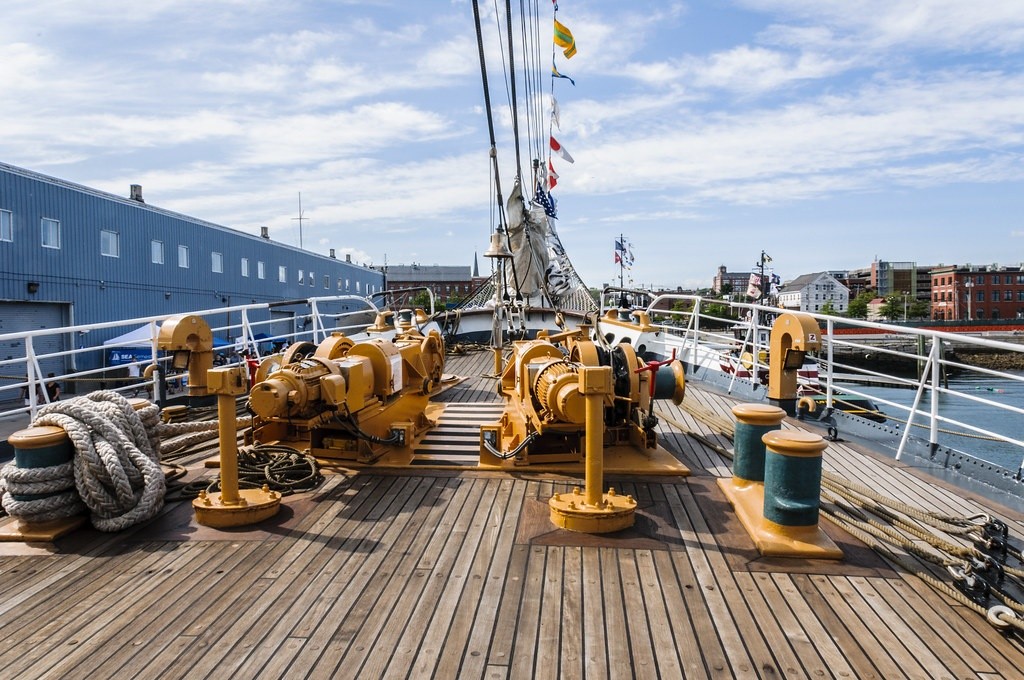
[
  {"xmin": 103, "ymin": 323, "xmax": 168, "ymax": 391},
  {"xmin": 213, "ymin": 336, "xmax": 232, "ymax": 365},
  {"xmin": 248, "ymin": 333, "xmax": 287, "ymax": 357}
]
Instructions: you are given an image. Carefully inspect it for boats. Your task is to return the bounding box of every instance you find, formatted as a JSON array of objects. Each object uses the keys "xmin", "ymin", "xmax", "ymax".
[{"xmin": 718, "ymin": 249, "xmax": 890, "ymax": 426}]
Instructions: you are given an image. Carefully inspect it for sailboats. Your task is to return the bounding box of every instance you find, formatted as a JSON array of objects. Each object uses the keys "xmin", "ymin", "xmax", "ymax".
[{"xmin": 428, "ymin": 0, "xmax": 605, "ymax": 345}]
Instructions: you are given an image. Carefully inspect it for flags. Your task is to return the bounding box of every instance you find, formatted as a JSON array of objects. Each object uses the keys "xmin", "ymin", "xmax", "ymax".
[
  {"xmin": 527, "ymin": 0, "xmax": 577, "ymax": 221},
  {"xmin": 614, "ymin": 240, "xmax": 634, "ymax": 285},
  {"xmin": 747, "ymin": 284, "xmax": 762, "ymax": 299},
  {"xmin": 750, "ymin": 273, "xmax": 760, "ymax": 285}
]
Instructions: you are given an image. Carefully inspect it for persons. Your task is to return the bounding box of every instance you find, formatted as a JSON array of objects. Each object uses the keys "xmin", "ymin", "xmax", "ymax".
[
  {"xmin": 270, "ymin": 347, "xmax": 278, "ymax": 355},
  {"xmin": 144, "ymin": 377, "xmax": 153, "ymax": 399},
  {"xmin": 127, "ymin": 356, "xmax": 142, "ymax": 398},
  {"xmin": 25, "ymin": 373, "xmax": 61, "ymax": 416},
  {"xmin": 213, "ymin": 353, "xmax": 227, "ymax": 367},
  {"xmin": 230, "ymin": 350, "xmax": 250, "ymax": 364}
]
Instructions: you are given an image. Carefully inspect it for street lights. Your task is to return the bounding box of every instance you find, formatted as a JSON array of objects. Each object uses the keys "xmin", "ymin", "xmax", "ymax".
[
  {"xmin": 965, "ymin": 276, "xmax": 975, "ymax": 321},
  {"xmin": 903, "ymin": 291, "xmax": 909, "ymax": 323}
]
